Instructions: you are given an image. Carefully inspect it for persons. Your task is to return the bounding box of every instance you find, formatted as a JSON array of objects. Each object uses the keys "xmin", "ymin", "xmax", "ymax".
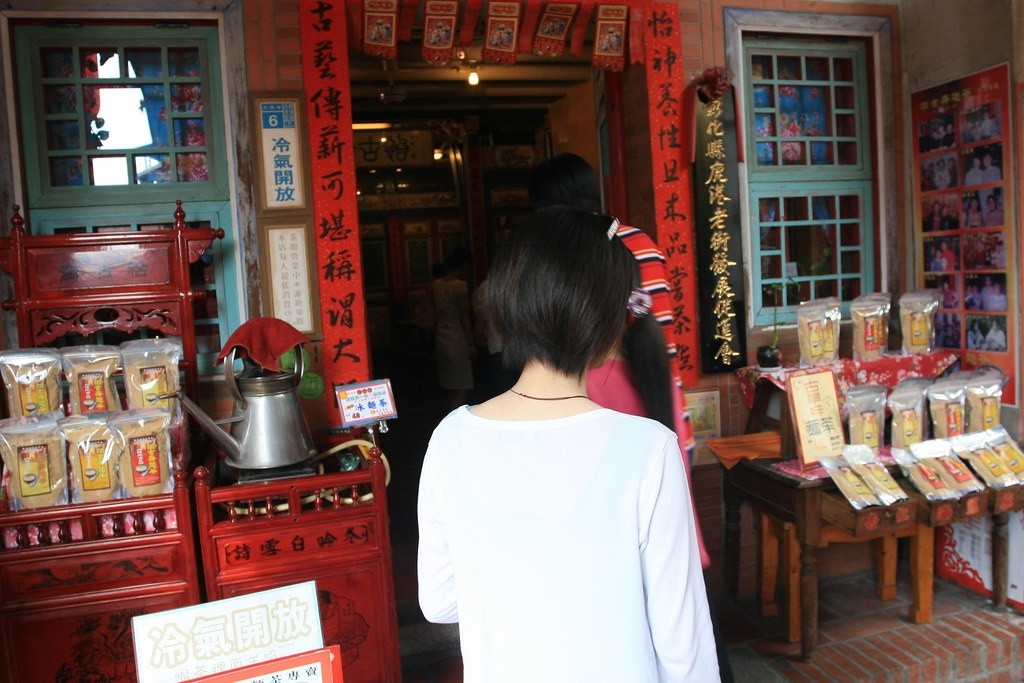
[
  {"xmin": 919, "ymin": 121, "xmax": 955, "ymax": 153},
  {"xmin": 936, "ymin": 282, "xmax": 958, "ymax": 308},
  {"xmin": 928, "ymin": 241, "xmax": 955, "ymax": 272},
  {"xmin": 935, "ymin": 319, "xmax": 960, "ymax": 347},
  {"xmin": 966, "ymin": 154, "xmax": 1001, "ymax": 185},
  {"xmin": 972, "ymin": 111, "xmax": 998, "ymax": 141},
  {"xmin": 968, "ymin": 318, "xmax": 1005, "ymax": 352},
  {"xmin": 520, "ymin": 153, "xmax": 714, "ymax": 566},
  {"xmin": 961, "ymin": 195, "xmax": 1006, "ymax": 228},
  {"xmin": 966, "ymin": 275, "xmax": 1008, "ymax": 311},
  {"xmin": 923, "ymin": 158, "xmax": 957, "ymax": 189},
  {"xmin": 416, "ymin": 204, "xmax": 725, "ymax": 683},
  {"xmin": 924, "ymin": 201, "xmax": 958, "ymax": 231}
]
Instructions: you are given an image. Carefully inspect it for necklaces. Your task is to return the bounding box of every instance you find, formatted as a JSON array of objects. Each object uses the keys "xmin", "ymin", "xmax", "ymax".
[{"xmin": 509, "ymin": 386, "xmax": 591, "ymax": 401}]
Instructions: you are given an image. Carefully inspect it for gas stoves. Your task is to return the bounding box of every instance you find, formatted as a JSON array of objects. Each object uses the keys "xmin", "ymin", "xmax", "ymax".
[{"xmin": 224, "ymin": 462, "xmax": 319, "ymax": 503}]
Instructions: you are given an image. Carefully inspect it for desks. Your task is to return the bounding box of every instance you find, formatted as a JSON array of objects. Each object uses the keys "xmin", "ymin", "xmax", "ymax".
[{"xmin": 702, "ymin": 425, "xmax": 1024, "ymax": 662}]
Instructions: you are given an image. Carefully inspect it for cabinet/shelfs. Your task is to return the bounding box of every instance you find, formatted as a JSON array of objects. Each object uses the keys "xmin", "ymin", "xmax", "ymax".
[
  {"xmin": 1, "ymin": 199, "xmax": 226, "ymax": 466},
  {"xmin": 0, "ymin": 459, "xmax": 200, "ymax": 683},
  {"xmin": 193, "ymin": 428, "xmax": 401, "ymax": 683}
]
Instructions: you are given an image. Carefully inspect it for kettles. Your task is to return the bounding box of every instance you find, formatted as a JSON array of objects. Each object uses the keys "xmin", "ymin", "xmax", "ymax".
[{"xmin": 158, "ymin": 318, "xmax": 317, "ymax": 471}]
